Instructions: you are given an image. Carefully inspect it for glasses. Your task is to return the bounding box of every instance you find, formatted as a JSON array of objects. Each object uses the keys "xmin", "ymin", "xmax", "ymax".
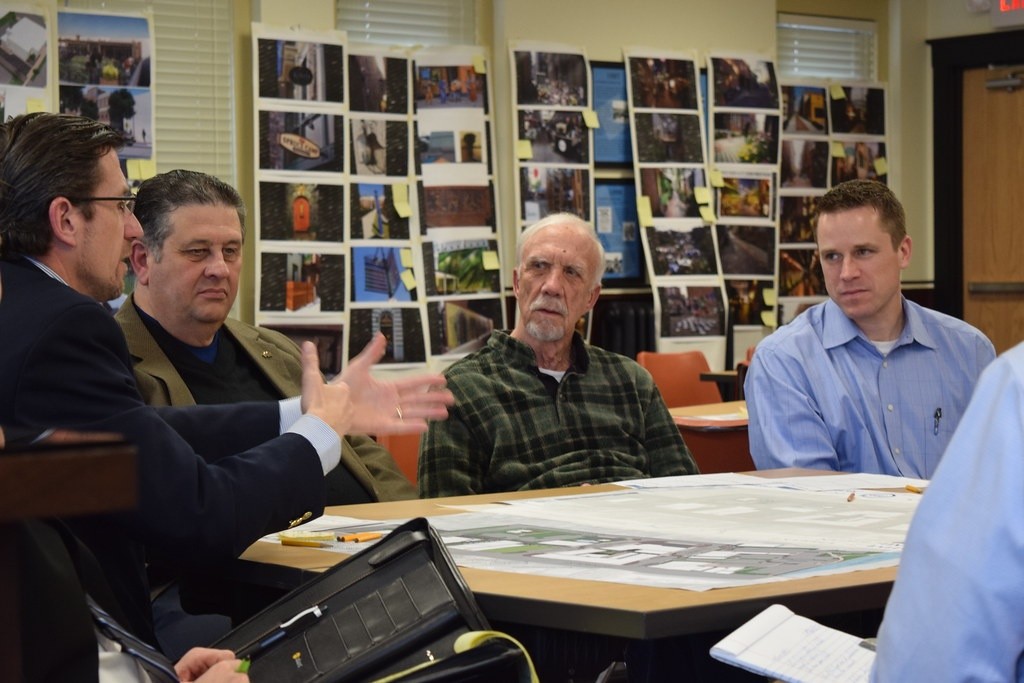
[{"xmin": 69, "ymin": 193, "xmax": 137, "ymax": 216}]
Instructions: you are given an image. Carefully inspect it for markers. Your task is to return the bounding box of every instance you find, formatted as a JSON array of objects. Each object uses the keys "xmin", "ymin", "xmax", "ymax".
[{"xmin": 337, "ymin": 533, "xmax": 383, "ymax": 543}]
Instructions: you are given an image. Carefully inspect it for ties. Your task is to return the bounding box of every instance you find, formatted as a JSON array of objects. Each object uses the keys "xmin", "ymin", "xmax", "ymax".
[{"xmin": 85, "ymin": 592, "xmax": 180, "ymax": 683}]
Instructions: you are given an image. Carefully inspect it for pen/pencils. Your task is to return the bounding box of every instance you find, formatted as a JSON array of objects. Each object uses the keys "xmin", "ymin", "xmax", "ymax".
[
  {"xmin": 847, "ymin": 493, "xmax": 855, "ymax": 502},
  {"xmin": 934, "ymin": 408, "xmax": 942, "ymax": 435},
  {"xmin": 906, "ymin": 485, "xmax": 921, "ymax": 494},
  {"xmin": 235, "ymin": 604, "xmax": 328, "ymax": 657},
  {"xmin": 282, "ymin": 539, "xmax": 334, "ymax": 549},
  {"xmin": 238, "ymin": 654, "xmax": 252, "ymax": 673}
]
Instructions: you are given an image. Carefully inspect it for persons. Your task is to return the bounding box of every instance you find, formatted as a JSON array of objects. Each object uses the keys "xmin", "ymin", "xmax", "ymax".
[
  {"xmin": 116, "ymin": 167, "xmax": 421, "ymax": 663},
  {"xmin": 871, "ymin": 343, "xmax": 1024, "ymax": 682},
  {"xmin": 0, "ymin": 111, "xmax": 458, "ymax": 683},
  {"xmin": 743, "ymin": 178, "xmax": 998, "ymax": 477},
  {"xmin": 417, "ymin": 212, "xmax": 701, "ymax": 498}
]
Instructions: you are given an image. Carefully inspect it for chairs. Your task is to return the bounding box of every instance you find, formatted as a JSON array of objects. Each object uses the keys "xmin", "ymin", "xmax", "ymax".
[{"xmin": 636, "ymin": 350, "xmax": 721, "ymax": 409}]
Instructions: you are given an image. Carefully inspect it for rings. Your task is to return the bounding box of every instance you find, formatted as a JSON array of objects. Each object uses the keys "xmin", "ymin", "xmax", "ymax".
[{"xmin": 397, "ymin": 405, "xmax": 403, "ymax": 420}]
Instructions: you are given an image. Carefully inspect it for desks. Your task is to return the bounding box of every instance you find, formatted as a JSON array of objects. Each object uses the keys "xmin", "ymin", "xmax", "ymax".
[
  {"xmin": 664, "ymin": 401, "xmax": 759, "ymax": 475},
  {"xmin": 238, "ymin": 466, "xmax": 929, "ymax": 683}
]
